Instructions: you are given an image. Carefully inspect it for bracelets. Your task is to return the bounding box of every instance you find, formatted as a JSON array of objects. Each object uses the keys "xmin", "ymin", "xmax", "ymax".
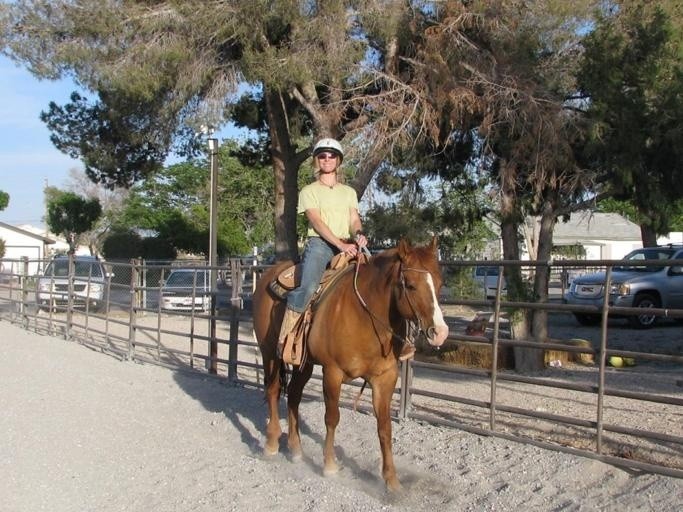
[{"xmin": 357, "ymin": 231, "xmax": 366, "ymax": 234}]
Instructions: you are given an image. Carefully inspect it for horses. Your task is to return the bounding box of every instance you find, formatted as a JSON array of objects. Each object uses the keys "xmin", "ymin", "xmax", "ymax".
[{"xmin": 250, "ymin": 234, "xmax": 449, "ymax": 493}]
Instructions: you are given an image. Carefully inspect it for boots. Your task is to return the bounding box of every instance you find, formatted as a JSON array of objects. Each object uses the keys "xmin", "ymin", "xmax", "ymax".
[{"xmin": 275, "ymin": 307, "xmax": 303, "ymax": 359}]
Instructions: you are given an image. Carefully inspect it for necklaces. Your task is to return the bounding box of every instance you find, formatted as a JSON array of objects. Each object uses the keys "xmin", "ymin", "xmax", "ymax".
[{"xmin": 320, "ymin": 179, "xmax": 337, "ymax": 189}]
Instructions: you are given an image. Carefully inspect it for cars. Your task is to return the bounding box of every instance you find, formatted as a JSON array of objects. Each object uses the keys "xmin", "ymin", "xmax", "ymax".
[
  {"xmin": 35, "ymin": 256, "xmax": 115, "ymax": 311},
  {"xmin": 564, "ymin": 245, "xmax": 683, "ymax": 330},
  {"xmin": 158, "ymin": 268, "xmax": 210, "ymax": 314},
  {"xmin": 474, "ymin": 266, "xmax": 506, "ymax": 299}
]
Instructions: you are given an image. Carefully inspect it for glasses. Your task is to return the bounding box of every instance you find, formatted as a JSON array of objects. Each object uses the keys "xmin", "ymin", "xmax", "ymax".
[{"xmin": 318, "ymin": 152, "xmax": 336, "ymax": 159}]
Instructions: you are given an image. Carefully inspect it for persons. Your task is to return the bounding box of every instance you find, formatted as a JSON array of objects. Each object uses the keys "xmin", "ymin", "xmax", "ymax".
[{"xmin": 276, "ymin": 138, "xmax": 369, "ymax": 361}]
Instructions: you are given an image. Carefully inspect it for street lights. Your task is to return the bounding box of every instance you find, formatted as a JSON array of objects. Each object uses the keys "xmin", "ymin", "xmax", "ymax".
[{"xmin": 200, "ymin": 124, "xmax": 218, "ymax": 375}]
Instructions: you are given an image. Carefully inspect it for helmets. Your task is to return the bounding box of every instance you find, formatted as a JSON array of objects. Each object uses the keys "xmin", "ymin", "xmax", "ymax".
[{"xmin": 313, "ymin": 137, "xmax": 344, "ymax": 162}]
